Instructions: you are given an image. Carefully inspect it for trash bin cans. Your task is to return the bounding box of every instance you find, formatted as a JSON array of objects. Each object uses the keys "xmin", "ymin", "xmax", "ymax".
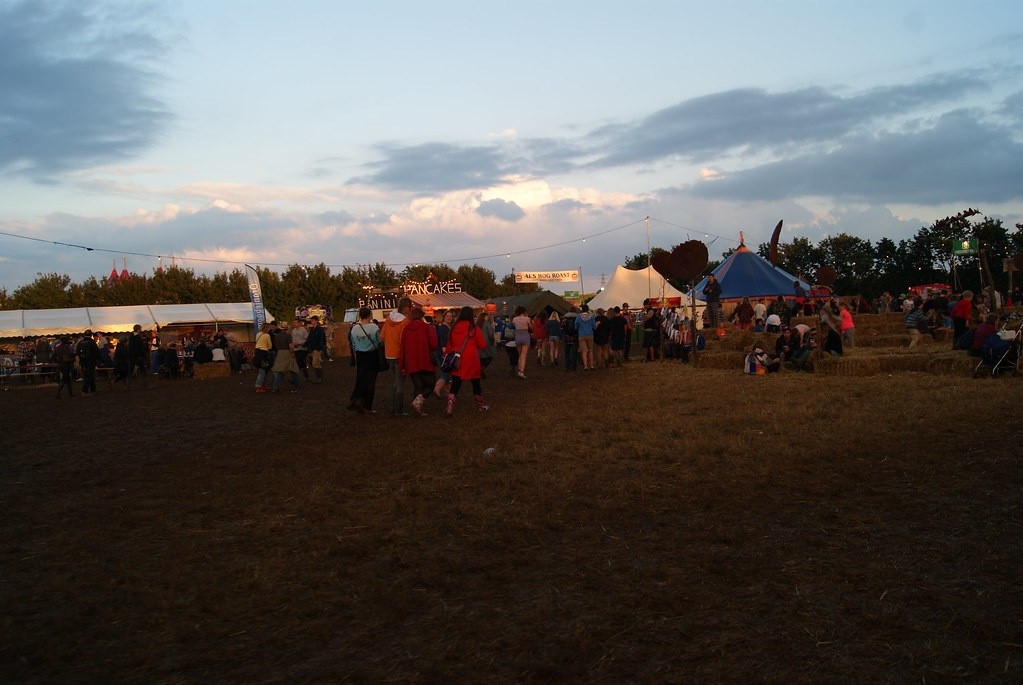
[{"xmin": 631, "ymin": 324, "xmax": 644, "ymax": 343}]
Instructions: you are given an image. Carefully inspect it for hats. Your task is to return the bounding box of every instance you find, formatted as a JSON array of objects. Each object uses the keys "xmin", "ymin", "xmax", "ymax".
[
  {"xmin": 309, "ymin": 315, "xmax": 319, "ymax": 321},
  {"xmin": 623, "ymin": 303, "xmax": 631, "ymax": 307},
  {"xmin": 278, "ymin": 321, "xmax": 290, "ymax": 330}
]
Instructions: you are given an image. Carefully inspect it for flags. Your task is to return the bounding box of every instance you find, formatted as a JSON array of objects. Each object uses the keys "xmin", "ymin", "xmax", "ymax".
[{"xmin": 245, "ymin": 263, "xmax": 266, "ymax": 335}]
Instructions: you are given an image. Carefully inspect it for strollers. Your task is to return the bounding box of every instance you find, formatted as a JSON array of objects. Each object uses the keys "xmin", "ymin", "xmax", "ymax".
[{"xmin": 973, "ymin": 321, "xmax": 1023, "ymax": 379}]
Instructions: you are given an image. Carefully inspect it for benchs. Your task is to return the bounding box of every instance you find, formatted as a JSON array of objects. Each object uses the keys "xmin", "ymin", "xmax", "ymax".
[{"xmin": 0, "ymin": 371, "xmax": 55, "ymax": 378}]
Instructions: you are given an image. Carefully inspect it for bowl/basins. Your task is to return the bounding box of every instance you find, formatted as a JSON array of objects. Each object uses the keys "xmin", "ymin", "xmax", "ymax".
[{"xmin": 1001, "ymin": 331, "xmax": 1015, "ymax": 337}]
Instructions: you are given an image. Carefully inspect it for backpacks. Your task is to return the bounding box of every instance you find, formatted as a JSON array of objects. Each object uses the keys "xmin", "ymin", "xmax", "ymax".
[{"xmin": 695, "ymin": 334, "xmax": 705, "ymax": 351}]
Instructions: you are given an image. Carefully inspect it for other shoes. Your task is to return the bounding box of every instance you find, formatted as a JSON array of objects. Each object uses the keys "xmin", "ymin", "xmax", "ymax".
[
  {"xmin": 112, "ymin": 380, "xmax": 116, "ymax": 388},
  {"xmin": 350, "ymin": 398, "xmax": 376, "ymax": 416},
  {"xmin": 55, "ymin": 394, "xmax": 65, "ymax": 399},
  {"xmin": 255, "ymin": 384, "xmax": 271, "ymax": 393},
  {"xmin": 535, "ymin": 357, "xmax": 632, "ymax": 372},
  {"xmin": 509, "ymin": 367, "xmax": 527, "ymax": 379},
  {"xmin": 328, "ymin": 358, "xmax": 335, "ymax": 362},
  {"xmin": 641, "ymin": 358, "xmax": 647, "ymax": 364},
  {"xmin": 81, "ymin": 391, "xmax": 96, "ymax": 397},
  {"xmin": 388, "ymin": 411, "xmax": 409, "ymax": 416},
  {"xmin": 68, "ymin": 392, "xmax": 73, "ymax": 397},
  {"xmin": 123, "ymin": 385, "xmax": 128, "ymax": 391}
]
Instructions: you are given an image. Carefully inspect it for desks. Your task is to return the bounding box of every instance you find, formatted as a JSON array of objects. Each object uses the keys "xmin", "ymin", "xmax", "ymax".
[{"xmin": 0, "ymin": 363, "xmax": 61, "ymax": 387}]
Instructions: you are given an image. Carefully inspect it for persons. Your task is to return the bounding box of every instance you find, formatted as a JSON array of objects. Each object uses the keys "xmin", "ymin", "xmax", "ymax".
[
  {"xmin": 0, "ymin": 312, "xmax": 334, "ymax": 398},
  {"xmin": 702, "ymin": 274, "xmax": 1023, "ymax": 376},
  {"xmin": 347, "ymin": 298, "xmax": 658, "ymax": 415}
]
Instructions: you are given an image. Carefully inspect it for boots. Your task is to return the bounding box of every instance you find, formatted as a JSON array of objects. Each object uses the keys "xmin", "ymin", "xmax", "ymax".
[
  {"xmin": 312, "ymin": 368, "xmax": 324, "ymax": 384},
  {"xmin": 433, "ymin": 378, "xmax": 451, "ymax": 398},
  {"xmin": 443, "ymin": 393, "xmax": 457, "ymax": 416},
  {"xmin": 301, "ymin": 367, "xmax": 310, "ymax": 383},
  {"xmin": 475, "ymin": 393, "xmax": 490, "ymax": 412},
  {"xmin": 413, "ymin": 393, "xmax": 428, "ymax": 417}
]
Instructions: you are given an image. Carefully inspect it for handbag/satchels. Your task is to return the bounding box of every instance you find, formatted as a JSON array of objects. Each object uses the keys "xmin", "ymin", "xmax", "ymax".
[
  {"xmin": 375, "ymin": 348, "xmax": 390, "ymax": 372},
  {"xmin": 441, "ymin": 350, "xmax": 461, "ymax": 373}
]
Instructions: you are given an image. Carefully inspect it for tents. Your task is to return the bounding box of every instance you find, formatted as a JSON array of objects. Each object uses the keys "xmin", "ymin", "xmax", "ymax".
[
  {"xmin": 586, "ymin": 264, "xmax": 707, "ymax": 330},
  {"xmin": 685, "ymin": 241, "xmax": 811, "ymax": 320},
  {"xmin": 0, "ymin": 302, "xmax": 275, "ymax": 338},
  {"xmin": 495, "ymin": 290, "xmax": 581, "ymax": 318}
]
